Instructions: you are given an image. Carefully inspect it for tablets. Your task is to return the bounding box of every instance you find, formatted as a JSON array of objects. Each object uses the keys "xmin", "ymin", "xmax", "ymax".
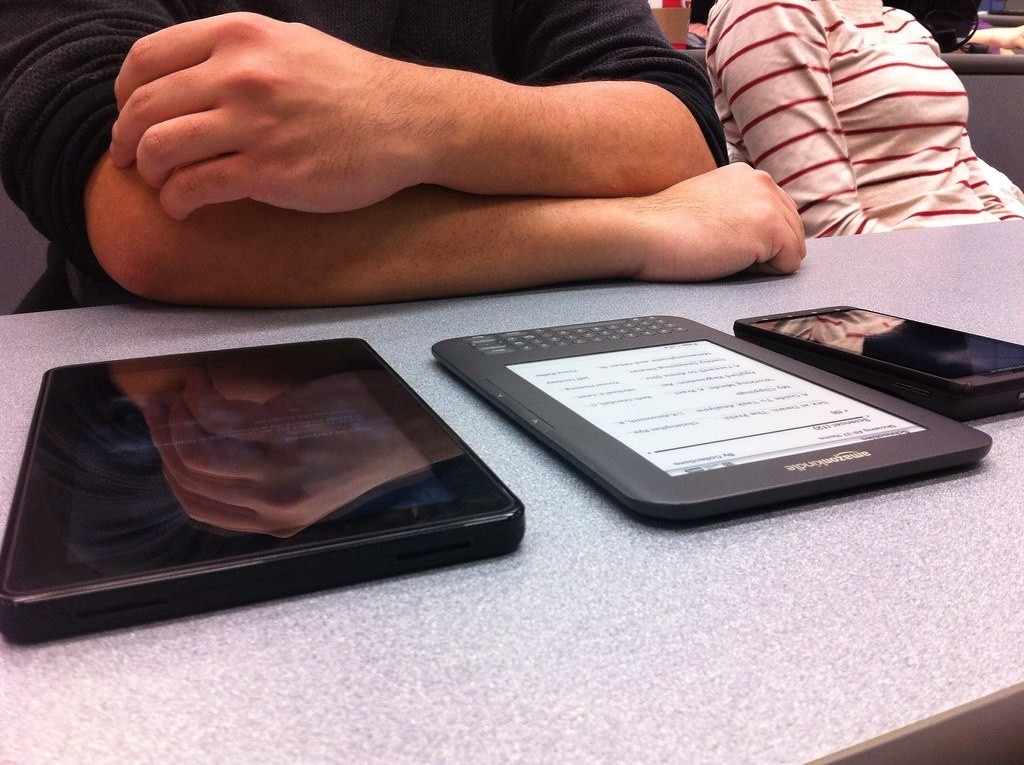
[
  {"xmin": 432, "ymin": 316, "xmax": 993, "ymax": 529},
  {"xmin": 0, "ymin": 338, "xmax": 526, "ymax": 645}
]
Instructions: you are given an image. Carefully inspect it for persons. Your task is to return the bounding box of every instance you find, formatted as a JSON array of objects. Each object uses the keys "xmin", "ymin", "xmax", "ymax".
[
  {"xmin": 45, "ymin": 340, "xmax": 473, "ymax": 577},
  {"xmin": 703, "ymin": 0, "xmax": 1024, "ymax": 239},
  {"xmin": 1, "ymin": 0, "xmax": 807, "ymax": 312},
  {"xmin": 751, "ymin": 308, "xmax": 906, "ymax": 357}
]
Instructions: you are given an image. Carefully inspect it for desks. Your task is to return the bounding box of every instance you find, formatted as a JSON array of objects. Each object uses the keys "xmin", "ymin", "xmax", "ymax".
[{"xmin": 0, "ymin": 222, "xmax": 1024, "ymax": 765}]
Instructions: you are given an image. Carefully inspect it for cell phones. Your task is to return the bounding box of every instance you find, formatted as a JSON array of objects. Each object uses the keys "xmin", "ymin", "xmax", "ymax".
[{"xmin": 733, "ymin": 305, "xmax": 1024, "ymax": 421}]
